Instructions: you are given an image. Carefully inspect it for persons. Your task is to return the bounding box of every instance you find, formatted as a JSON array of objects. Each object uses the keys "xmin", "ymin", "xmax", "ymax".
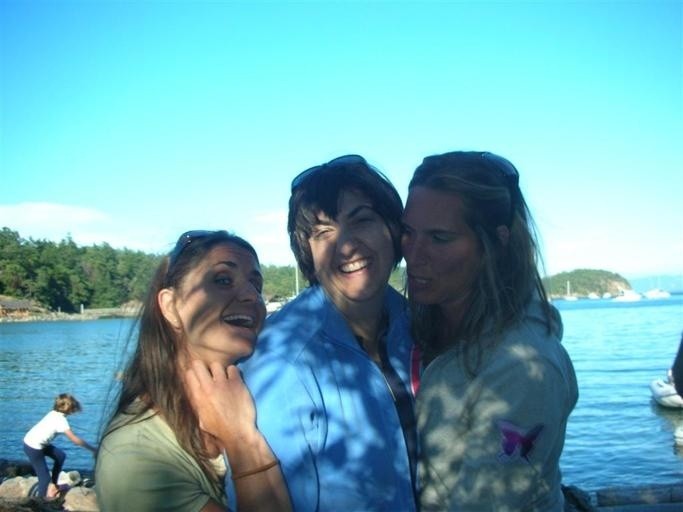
[
  {"xmin": 222, "ymin": 152, "xmax": 430, "ymax": 512},
  {"xmin": 92, "ymin": 228, "xmax": 293, "ymax": 512},
  {"xmin": 400, "ymin": 148, "xmax": 581, "ymax": 512},
  {"xmin": 21, "ymin": 392, "xmax": 100, "ymax": 512}
]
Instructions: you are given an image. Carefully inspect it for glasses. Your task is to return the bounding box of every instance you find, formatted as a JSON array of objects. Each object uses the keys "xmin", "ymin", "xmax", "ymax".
[
  {"xmin": 426, "ymin": 152, "xmax": 518, "ymax": 184},
  {"xmin": 288, "ymin": 154, "xmax": 365, "ymax": 194},
  {"xmin": 167, "ymin": 229, "xmax": 217, "ymax": 280}
]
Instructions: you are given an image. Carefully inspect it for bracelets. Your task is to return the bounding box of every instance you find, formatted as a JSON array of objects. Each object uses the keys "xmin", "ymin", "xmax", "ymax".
[{"xmin": 230, "ymin": 458, "xmax": 282, "ymax": 480}]
